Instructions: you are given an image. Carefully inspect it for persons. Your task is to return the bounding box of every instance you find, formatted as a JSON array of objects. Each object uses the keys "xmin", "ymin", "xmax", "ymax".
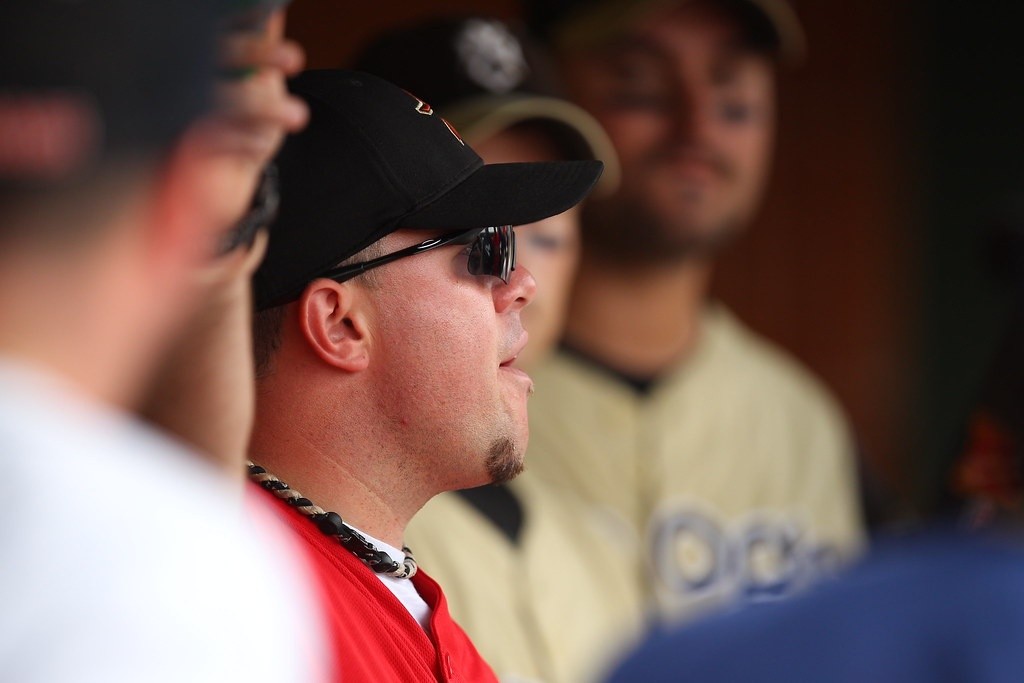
[
  {"xmin": 529, "ymin": 0, "xmax": 875, "ymax": 637},
  {"xmin": 241, "ymin": 65, "xmax": 603, "ymax": 683},
  {"xmin": 0, "ymin": 1, "xmax": 336, "ymax": 683},
  {"xmin": 352, "ymin": 20, "xmax": 641, "ymax": 681}
]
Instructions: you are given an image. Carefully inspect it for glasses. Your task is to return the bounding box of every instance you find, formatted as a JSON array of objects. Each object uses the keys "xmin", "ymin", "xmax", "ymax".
[
  {"xmin": 257, "ymin": 223, "xmax": 517, "ymax": 285},
  {"xmin": 205, "ymin": 176, "xmax": 282, "ymax": 251}
]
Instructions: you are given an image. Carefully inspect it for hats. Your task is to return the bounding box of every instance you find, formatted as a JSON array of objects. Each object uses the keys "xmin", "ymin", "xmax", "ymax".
[
  {"xmin": 352, "ymin": 15, "xmax": 622, "ymax": 209},
  {"xmin": 255, "ymin": 67, "xmax": 604, "ymax": 295},
  {"xmin": 520, "ymin": 2, "xmax": 810, "ymax": 73}
]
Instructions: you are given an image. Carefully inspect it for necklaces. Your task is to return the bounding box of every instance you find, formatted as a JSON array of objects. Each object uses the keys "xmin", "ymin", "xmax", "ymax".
[{"xmin": 242, "ymin": 459, "xmax": 418, "ymax": 578}]
